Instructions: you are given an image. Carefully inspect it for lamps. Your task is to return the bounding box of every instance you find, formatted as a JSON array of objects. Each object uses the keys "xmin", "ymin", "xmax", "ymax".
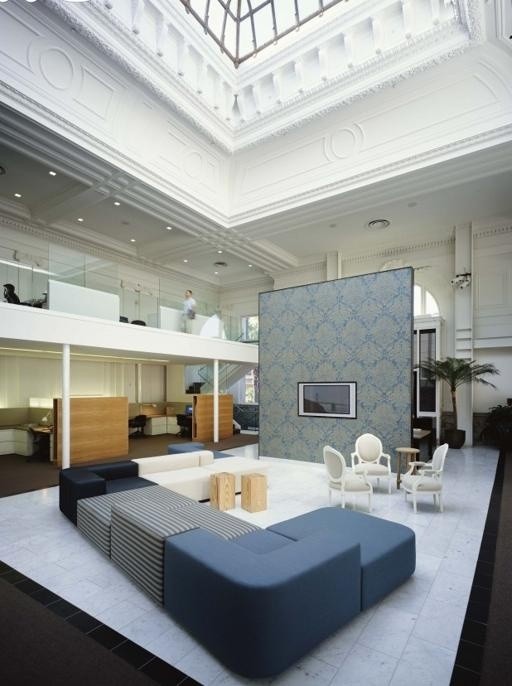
[{"xmin": 449, "ymin": 272, "xmax": 471, "ymax": 290}]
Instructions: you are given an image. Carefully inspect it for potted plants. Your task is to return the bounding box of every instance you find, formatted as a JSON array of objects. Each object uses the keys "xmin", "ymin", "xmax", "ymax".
[{"xmin": 414, "ymin": 356, "xmax": 501, "ymax": 450}]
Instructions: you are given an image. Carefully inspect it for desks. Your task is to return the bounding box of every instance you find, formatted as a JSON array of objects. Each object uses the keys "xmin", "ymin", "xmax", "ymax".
[
  {"xmin": 0, "ymin": 425, "xmax": 53, "ymax": 462},
  {"xmin": 145, "ymin": 415, "xmax": 180, "ymax": 436},
  {"xmin": 412, "ymin": 429, "xmax": 433, "ymax": 462}
]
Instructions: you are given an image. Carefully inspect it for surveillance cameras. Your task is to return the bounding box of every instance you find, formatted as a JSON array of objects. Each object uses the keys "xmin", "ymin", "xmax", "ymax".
[
  {"xmin": 459, "ymin": 282, "xmax": 468, "ymax": 291},
  {"xmin": 449, "ymin": 277, "xmax": 461, "ymax": 288}
]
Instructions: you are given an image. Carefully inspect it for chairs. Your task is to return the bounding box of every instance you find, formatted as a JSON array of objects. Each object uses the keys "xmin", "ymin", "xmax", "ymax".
[
  {"xmin": 129, "ymin": 415, "xmax": 147, "ymax": 439},
  {"xmin": 26, "ymin": 427, "xmax": 48, "ymax": 462},
  {"xmin": 175, "ymin": 414, "xmax": 189, "ymax": 438},
  {"xmin": 322, "ymin": 432, "xmax": 450, "ymax": 512},
  {"xmin": 3, "ymin": 282, "xmax": 31, "ymax": 306}
]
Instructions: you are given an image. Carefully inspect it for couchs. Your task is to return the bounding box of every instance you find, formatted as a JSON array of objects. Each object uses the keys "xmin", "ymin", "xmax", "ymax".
[{"xmin": 57, "ymin": 449, "xmax": 417, "ymax": 680}]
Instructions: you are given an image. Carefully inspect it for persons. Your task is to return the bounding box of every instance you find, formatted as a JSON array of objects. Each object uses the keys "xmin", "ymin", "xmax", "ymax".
[
  {"xmin": 2, "ymin": 283, "xmax": 20, "ymax": 303},
  {"xmin": 181, "ymin": 289, "xmax": 196, "ymax": 335}
]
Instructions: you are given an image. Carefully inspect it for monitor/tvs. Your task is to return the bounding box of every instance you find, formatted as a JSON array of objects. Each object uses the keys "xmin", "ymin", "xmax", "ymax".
[{"xmin": 186, "ymin": 405, "xmax": 193, "ymax": 414}]
